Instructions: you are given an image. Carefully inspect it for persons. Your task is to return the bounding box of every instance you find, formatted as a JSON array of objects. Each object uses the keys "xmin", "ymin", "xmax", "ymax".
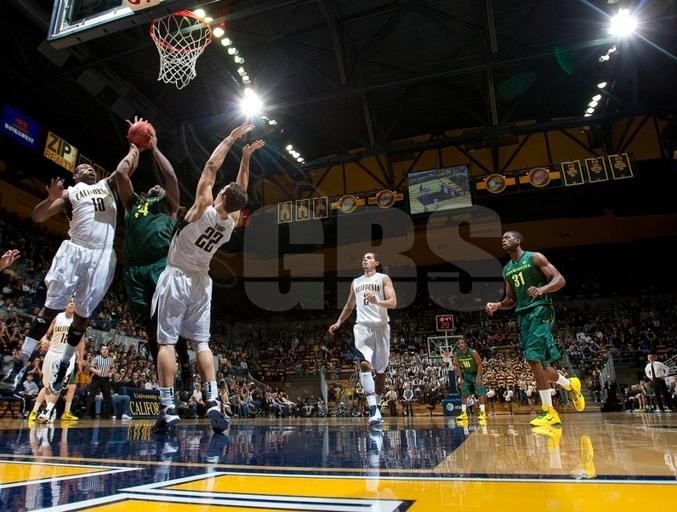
[
  {"xmin": 0, "ymin": 424, "xmax": 677, "ymax": 512},
  {"xmin": 150, "ymin": 121, "xmax": 265, "ymax": 433},
  {"xmin": 417, "ymin": 180, "xmax": 466, "ymax": 215},
  {"xmin": 2, "ymin": 116, "xmax": 152, "ymax": 393},
  {"xmin": 116, "ymin": 126, "xmax": 194, "ymax": 377},
  {"xmin": 0, "ymin": 231, "xmax": 676, "ymax": 420}
]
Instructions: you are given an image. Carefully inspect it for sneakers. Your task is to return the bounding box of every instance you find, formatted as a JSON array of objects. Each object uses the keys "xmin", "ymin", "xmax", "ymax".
[
  {"xmin": 150, "ymin": 403, "xmax": 180, "ymax": 435},
  {"xmin": 563, "ymin": 377, "xmax": 585, "ymax": 412},
  {"xmin": 204, "ymin": 398, "xmax": 229, "ymax": 432},
  {"xmin": 0, "ymin": 356, "xmax": 32, "ymax": 391},
  {"xmin": 456, "ymin": 411, "xmax": 468, "ymax": 420},
  {"xmin": 478, "ymin": 411, "xmax": 487, "ymax": 419},
  {"xmin": 368, "ymin": 405, "xmax": 384, "ymax": 425},
  {"xmin": 18, "ymin": 410, "xmax": 132, "ymax": 422},
  {"xmin": 530, "ymin": 404, "xmax": 561, "ymax": 426},
  {"xmin": 48, "ymin": 357, "xmax": 70, "ymax": 395}
]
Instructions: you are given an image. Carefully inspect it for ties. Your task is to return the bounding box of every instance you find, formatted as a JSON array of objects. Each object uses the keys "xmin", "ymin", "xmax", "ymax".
[{"xmin": 651, "ymin": 363, "xmax": 655, "ymax": 379}]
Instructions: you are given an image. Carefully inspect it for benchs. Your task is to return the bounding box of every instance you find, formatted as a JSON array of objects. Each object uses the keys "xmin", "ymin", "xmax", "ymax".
[{"xmin": 0, "ymin": 396, "xmax": 21, "ymax": 419}]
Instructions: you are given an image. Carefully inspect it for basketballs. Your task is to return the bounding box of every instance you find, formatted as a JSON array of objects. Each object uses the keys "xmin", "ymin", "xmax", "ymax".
[{"xmin": 128, "ymin": 123, "xmax": 155, "ymax": 148}]
